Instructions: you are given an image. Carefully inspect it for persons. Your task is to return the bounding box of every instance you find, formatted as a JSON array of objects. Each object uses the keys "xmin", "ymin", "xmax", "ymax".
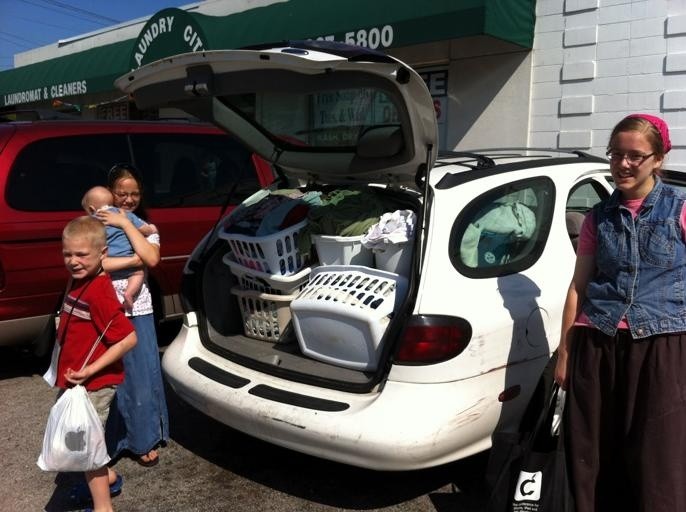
[
  {"xmin": 553, "ymin": 113, "xmax": 686, "ymax": 512},
  {"xmin": 53, "ymin": 216, "xmax": 137, "ymax": 512},
  {"xmin": 81, "ymin": 187, "xmax": 159, "ymax": 312},
  {"xmin": 97, "ymin": 162, "xmax": 170, "ymax": 468}
]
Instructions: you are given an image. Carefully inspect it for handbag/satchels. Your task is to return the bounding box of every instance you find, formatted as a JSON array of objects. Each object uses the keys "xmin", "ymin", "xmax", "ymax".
[{"xmin": 491, "ymin": 382, "xmax": 574, "ymax": 511}]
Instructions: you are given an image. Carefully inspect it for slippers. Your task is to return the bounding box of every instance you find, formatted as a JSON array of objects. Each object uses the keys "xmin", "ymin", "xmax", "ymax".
[
  {"xmin": 67, "ymin": 476, "xmax": 123, "ymax": 501},
  {"xmin": 137, "ymin": 453, "xmax": 158, "ymax": 467}
]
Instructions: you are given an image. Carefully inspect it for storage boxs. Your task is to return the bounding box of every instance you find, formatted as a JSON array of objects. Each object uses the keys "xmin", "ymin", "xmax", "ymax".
[
  {"xmin": 375, "ymin": 238, "xmax": 414, "ymax": 275},
  {"xmin": 311, "ymin": 233, "xmax": 375, "ymax": 268}
]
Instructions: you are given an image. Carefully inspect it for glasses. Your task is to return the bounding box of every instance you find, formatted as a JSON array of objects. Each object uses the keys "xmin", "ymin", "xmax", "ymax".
[{"xmin": 606, "ymin": 149, "xmax": 656, "ymax": 162}]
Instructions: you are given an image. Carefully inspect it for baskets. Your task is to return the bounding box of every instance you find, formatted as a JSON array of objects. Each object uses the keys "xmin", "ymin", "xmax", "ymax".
[{"xmin": 218, "ymin": 219, "xmax": 409, "ymax": 372}]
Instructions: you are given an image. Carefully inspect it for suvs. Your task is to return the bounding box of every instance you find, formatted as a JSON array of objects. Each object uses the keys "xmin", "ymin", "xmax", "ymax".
[
  {"xmin": 114, "ymin": 41, "xmax": 684, "ymax": 478},
  {"xmin": 2, "ymin": 123, "xmax": 274, "ymax": 315}
]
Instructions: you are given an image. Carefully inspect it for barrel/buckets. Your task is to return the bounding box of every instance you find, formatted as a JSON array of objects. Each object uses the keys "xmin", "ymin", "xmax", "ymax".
[{"xmin": 373, "ymin": 239, "xmax": 413, "ymax": 279}]
